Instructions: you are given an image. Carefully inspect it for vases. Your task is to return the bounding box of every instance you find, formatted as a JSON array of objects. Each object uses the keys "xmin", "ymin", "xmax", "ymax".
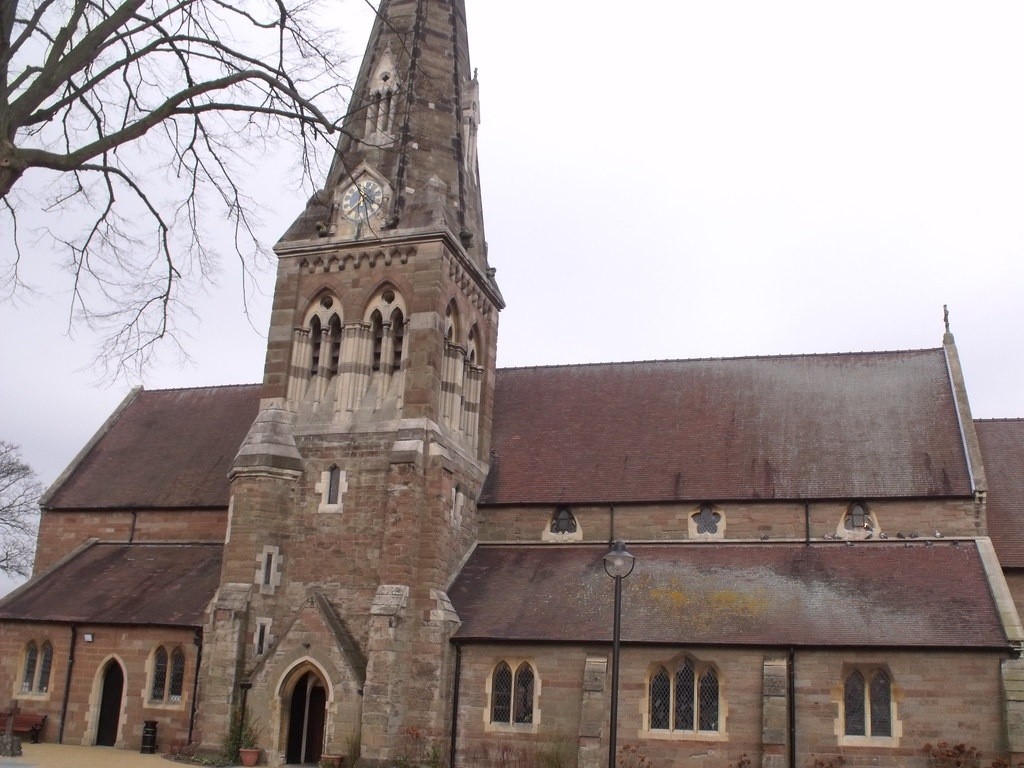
[{"xmin": 320, "ymin": 753, "xmax": 342, "ymax": 768}]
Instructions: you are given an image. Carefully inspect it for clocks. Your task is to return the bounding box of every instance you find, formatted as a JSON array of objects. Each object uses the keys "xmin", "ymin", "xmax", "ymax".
[{"xmin": 341, "ymin": 180, "xmax": 384, "ymax": 220}]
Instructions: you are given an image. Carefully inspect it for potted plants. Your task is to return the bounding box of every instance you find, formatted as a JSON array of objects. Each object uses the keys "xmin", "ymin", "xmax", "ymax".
[{"xmin": 239, "ymin": 725, "xmax": 262, "ymax": 767}]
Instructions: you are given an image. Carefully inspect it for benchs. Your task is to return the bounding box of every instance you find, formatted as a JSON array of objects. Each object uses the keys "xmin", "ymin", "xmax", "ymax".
[{"xmin": 0, "ymin": 714, "xmax": 48, "ymax": 744}]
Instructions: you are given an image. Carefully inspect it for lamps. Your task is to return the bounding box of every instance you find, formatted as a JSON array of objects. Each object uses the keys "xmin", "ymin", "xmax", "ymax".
[{"xmin": 84, "ymin": 633, "xmax": 94, "ymax": 642}]
